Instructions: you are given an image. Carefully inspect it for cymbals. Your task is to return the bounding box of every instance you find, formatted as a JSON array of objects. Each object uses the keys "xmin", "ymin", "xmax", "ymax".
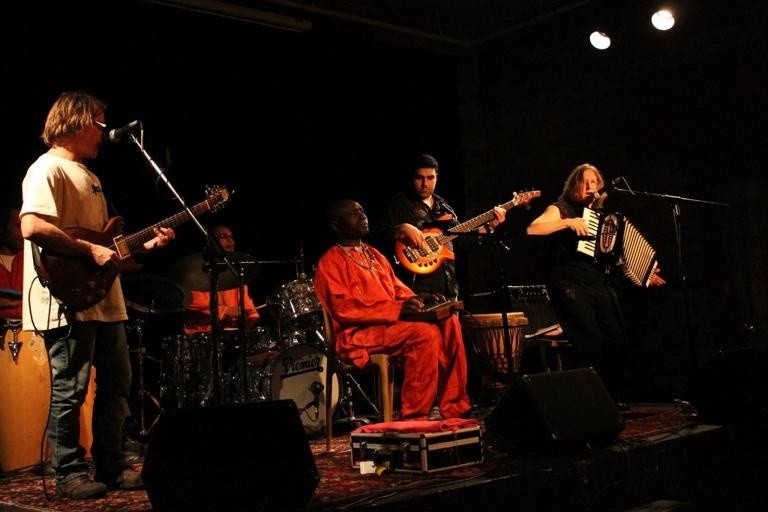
[
  {"xmin": 171, "ymin": 249, "xmax": 259, "ymax": 292},
  {"xmin": 118, "ymin": 275, "xmax": 184, "ymax": 313}
]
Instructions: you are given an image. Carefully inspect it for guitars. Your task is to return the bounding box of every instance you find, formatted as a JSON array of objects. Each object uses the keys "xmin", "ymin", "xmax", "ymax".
[
  {"xmin": 32, "ymin": 183, "xmax": 235, "ymax": 312},
  {"xmin": 396, "ymin": 187, "xmax": 541, "ymax": 273}
]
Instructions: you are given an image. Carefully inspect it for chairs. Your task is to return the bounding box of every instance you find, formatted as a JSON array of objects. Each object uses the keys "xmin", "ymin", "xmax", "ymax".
[{"xmin": 322, "ymin": 305, "xmax": 397, "ymax": 452}]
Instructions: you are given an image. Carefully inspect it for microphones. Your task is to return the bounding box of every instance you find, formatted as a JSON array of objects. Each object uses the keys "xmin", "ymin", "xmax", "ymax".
[
  {"xmin": 109, "ymin": 120, "xmax": 142, "ymax": 138},
  {"xmin": 595, "ymin": 181, "xmax": 617, "ymax": 200}
]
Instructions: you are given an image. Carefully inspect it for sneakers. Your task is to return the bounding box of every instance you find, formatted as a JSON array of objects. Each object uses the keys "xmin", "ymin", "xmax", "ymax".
[
  {"xmin": 116, "ymin": 468, "xmax": 143, "ymax": 489},
  {"xmin": 57, "ymin": 473, "xmax": 108, "ymax": 499}
]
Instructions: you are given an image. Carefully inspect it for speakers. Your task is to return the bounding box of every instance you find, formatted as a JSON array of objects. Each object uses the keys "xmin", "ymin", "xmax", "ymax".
[
  {"xmin": 137, "ymin": 397, "xmax": 320, "ymax": 512},
  {"xmin": 491, "ymin": 365, "xmax": 623, "ymax": 464}
]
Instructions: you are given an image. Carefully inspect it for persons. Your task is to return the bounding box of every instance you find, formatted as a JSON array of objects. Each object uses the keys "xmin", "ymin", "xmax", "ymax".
[
  {"xmin": 19, "ymin": 90, "xmax": 176, "ymax": 500},
  {"xmin": 525, "ymin": 163, "xmax": 667, "ymax": 411},
  {"xmin": 314, "ymin": 199, "xmax": 472, "ymax": 469},
  {"xmin": 377, "ymin": 153, "xmax": 507, "ymax": 318},
  {"xmin": 0, "ymin": 208, "xmax": 24, "ymax": 319},
  {"xmin": 183, "ymin": 225, "xmax": 263, "ymax": 337}
]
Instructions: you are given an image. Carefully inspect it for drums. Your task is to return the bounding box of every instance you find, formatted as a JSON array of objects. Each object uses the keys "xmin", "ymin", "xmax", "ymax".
[
  {"xmin": 264, "ymin": 277, "xmax": 323, "ymax": 328},
  {"xmin": 242, "ymin": 303, "xmax": 304, "ymax": 354},
  {"xmin": 0, "ymin": 318, "xmax": 97, "ymax": 473},
  {"xmin": 233, "ymin": 342, "xmax": 345, "ymax": 436},
  {"xmin": 461, "ymin": 312, "xmax": 528, "ymax": 384}
]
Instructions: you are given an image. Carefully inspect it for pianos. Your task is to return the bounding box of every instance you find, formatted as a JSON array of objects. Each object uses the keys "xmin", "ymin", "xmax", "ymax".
[{"xmin": 401, "ymin": 295, "xmax": 465, "ymax": 323}]
